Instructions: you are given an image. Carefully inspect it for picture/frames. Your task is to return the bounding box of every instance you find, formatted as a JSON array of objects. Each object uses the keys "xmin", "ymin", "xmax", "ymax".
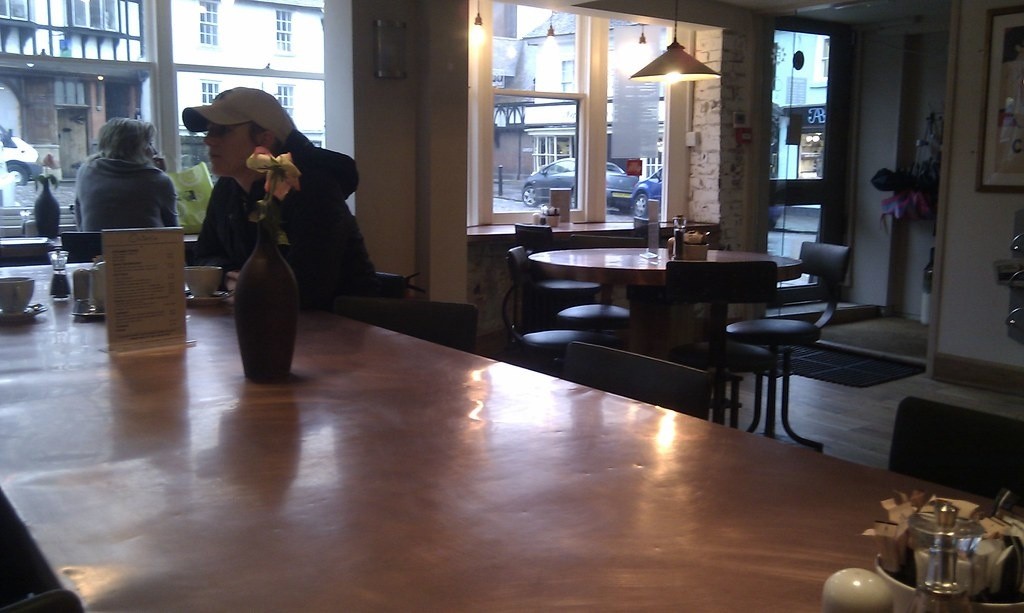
[{"xmin": 975, "ymin": 5, "xmax": 1024, "ymax": 194}]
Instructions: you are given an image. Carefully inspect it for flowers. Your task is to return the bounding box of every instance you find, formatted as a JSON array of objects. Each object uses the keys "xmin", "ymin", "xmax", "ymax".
[
  {"xmin": 246, "ymin": 146, "xmax": 303, "ymax": 245},
  {"xmin": 31, "ymin": 153, "xmax": 58, "ymax": 190}
]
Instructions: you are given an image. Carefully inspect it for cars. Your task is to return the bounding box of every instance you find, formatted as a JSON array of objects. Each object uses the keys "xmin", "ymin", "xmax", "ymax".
[{"xmin": 522, "ymin": 157, "xmax": 662, "ymax": 219}]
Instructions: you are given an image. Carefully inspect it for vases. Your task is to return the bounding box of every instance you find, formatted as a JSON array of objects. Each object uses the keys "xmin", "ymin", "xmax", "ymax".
[
  {"xmin": 34, "ymin": 178, "xmax": 60, "ymax": 239},
  {"xmin": 234, "ymin": 222, "xmax": 299, "ymax": 383}
]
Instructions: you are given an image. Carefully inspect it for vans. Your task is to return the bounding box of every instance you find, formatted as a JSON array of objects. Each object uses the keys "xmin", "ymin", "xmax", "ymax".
[{"xmin": 0, "ymin": 122, "xmax": 41, "ymax": 186}]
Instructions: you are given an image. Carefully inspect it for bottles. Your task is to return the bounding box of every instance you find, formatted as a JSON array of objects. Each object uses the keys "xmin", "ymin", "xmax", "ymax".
[
  {"xmin": 819, "ymin": 568, "xmax": 894, "ymax": 613},
  {"xmin": 540, "ymin": 204, "xmax": 548, "ymax": 225},
  {"xmin": 908, "ymin": 513, "xmax": 982, "ymax": 591},
  {"xmin": 907, "ymin": 580, "xmax": 973, "ymax": 613},
  {"xmin": 927, "ymin": 503, "xmax": 959, "ymax": 581},
  {"xmin": 74, "ymin": 269, "xmax": 92, "ymax": 314},
  {"xmin": 673, "ymin": 215, "xmax": 686, "ymax": 260},
  {"xmin": 48, "ymin": 251, "xmax": 70, "ymax": 300}
]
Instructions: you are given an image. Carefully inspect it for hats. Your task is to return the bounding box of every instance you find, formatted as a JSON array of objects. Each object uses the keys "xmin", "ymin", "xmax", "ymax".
[{"xmin": 182, "ymin": 87, "xmax": 293, "ymax": 140}]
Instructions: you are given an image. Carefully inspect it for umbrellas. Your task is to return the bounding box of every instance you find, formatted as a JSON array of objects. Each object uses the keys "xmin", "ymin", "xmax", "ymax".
[{"xmin": 871, "ymin": 139, "xmax": 933, "ymax": 224}]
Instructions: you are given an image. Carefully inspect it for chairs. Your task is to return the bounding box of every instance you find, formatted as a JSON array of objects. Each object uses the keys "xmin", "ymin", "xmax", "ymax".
[
  {"xmin": 627, "ymin": 260, "xmax": 778, "ymax": 425},
  {"xmin": 502, "ymin": 223, "xmax": 631, "ymax": 376},
  {"xmin": 334, "ymin": 295, "xmax": 478, "ymax": 354},
  {"xmin": 888, "ymin": 396, "xmax": 1024, "ymax": 507}
]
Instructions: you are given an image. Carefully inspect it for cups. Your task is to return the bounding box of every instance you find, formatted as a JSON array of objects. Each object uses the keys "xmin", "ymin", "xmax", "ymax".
[
  {"xmin": 668, "ymin": 238, "xmax": 675, "ymax": 260},
  {"xmin": 1, "ymin": 277, "xmax": 34, "ymax": 315},
  {"xmin": 184, "ymin": 267, "xmax": 222, "ymax": 297},
  {"xmin": 546, "ymin": 215, "xmax": 561, "ymax": 226},
  {"xmin": 533, "ymin": 213, "xmax": 540, "ymax": 225}
]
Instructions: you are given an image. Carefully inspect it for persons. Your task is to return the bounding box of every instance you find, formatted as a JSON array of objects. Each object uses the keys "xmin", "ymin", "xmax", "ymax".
[
  {"xmin": 182, "ymin": 87, "xmax": 377, "ymax": 309},
  {"xmin": 73, "ymin": 117, "xmax": 178, "ymax": 232}
]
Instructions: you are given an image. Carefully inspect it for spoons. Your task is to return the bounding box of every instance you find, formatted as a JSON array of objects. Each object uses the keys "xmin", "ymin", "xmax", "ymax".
[{"xmin": 27, "ymin": 304, "xmax": 45, "ymax": 311}]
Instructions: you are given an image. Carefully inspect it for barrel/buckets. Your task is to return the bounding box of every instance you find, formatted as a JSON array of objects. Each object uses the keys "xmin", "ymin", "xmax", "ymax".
[{"xmin": 873, "ymin": 554, "xmax": 1024, "ymax": 613}]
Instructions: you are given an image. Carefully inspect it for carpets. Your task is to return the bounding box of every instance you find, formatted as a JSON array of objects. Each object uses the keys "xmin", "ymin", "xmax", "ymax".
[{"xmin": 765, "ymin": 341, "xmax": 928, "ymax": 388}]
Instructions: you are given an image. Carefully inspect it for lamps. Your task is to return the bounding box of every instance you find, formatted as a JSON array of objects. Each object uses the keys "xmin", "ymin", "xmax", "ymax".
[{"xmin": 631, "ymin": 0, "xmax": 722, "ymax": 82}]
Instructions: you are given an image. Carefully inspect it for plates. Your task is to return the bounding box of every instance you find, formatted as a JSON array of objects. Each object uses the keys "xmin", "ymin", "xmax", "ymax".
[
  {"xmin": 0, "ymin": 308, "xmax": 34, "ymax": 323},
  {"xmin": 522, "ymin": 222, "xmax": 533, "ymax": 225},
  {"xmin": 184, "ymin": 290, "xmax": 232, "ymax": 305}
]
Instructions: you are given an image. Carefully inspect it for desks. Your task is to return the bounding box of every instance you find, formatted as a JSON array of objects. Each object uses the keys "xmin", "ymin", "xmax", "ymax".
[
  {"xmin": 0, "ymin": 263, "xmax": 1024, "ymax": 613},
  {"xmin": 528, "ymin": 248, "xmax": 802, "ymax": 425}
]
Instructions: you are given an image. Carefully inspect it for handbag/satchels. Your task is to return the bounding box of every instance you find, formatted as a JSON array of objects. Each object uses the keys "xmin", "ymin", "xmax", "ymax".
[{"xmin": 165, "ymin": 163, "xmax": 214, "ymax": 235}]
